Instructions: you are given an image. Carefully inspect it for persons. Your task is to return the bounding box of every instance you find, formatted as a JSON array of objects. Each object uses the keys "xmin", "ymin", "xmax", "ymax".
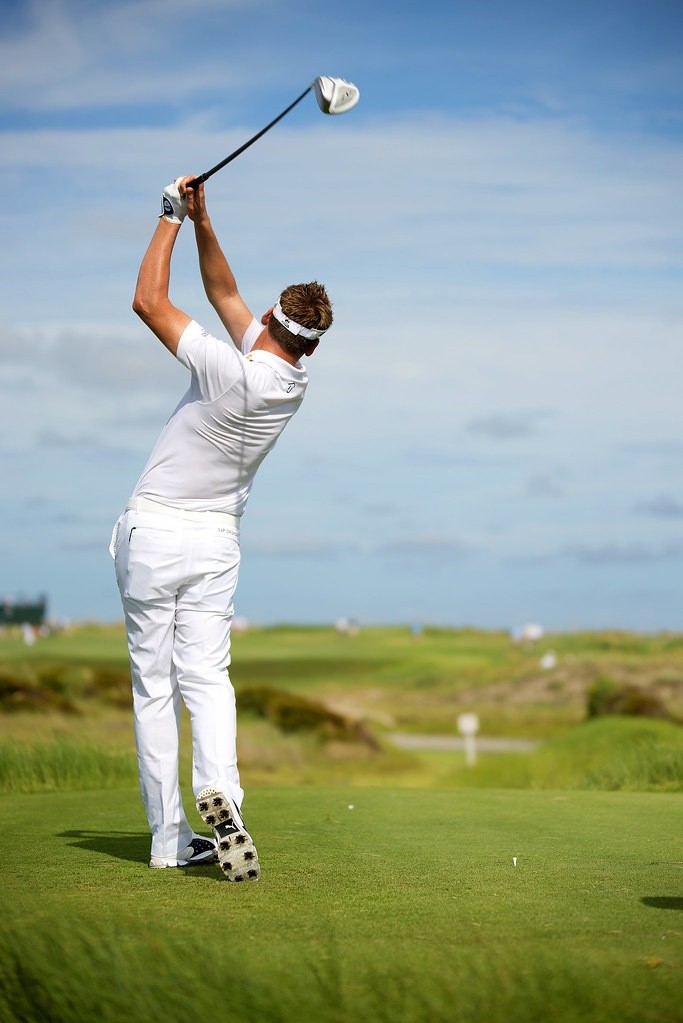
[{"xmin": 109, "ymin": 175, "xmax": 333, "ymax": 882}]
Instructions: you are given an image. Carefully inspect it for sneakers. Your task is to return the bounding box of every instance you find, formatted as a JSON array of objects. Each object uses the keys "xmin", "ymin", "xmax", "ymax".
[
  {"xmin": 195, "ymin": 788, "xmax": 262, "ymax": 881},
  {"xmin": 150, "ymin": 834, "xmax": 217, "ymax": 869}
]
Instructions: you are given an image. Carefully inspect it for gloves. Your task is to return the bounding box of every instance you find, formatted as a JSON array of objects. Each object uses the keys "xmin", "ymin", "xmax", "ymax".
[{"xmin": 159, "ymin": 176, "xmax": 188, "ymax": 224}]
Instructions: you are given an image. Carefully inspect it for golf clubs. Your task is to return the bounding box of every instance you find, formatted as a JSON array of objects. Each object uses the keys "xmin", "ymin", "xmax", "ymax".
[{"xmin": 184, "ymin": 75, "xmax": 361, "ymax": 196}]
